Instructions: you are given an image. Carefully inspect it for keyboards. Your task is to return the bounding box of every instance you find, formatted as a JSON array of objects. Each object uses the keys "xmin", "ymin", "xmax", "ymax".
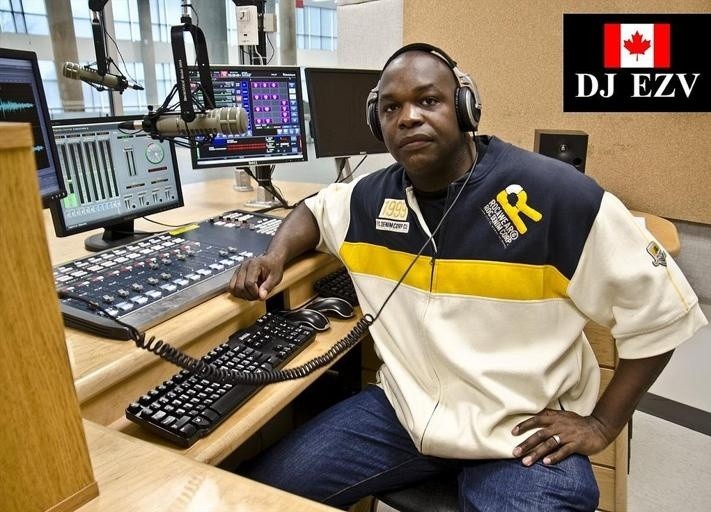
[
  {"xmin": 125, "ymin": 313, "xmax": 315, "ymax": 450},
  {"xmin": 314, "ymin": 267, "xmax": 360, "ymax": 308}
]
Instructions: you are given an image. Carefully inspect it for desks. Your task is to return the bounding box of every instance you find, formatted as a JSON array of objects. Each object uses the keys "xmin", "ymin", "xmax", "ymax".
[{"xmin": 37, "ymin": 177, "xmax": 364, "ymax": 512}]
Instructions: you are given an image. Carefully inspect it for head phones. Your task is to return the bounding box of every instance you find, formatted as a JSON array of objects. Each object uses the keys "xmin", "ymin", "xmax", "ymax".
[{"xmin": 366, "ymin": 43, "xmax": 479, "ymax": 143}]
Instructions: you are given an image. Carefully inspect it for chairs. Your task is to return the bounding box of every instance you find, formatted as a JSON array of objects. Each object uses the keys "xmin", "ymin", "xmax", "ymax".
[{"xmin": 346, "ymin": 206, "xmax": 680, "ymax": 512}]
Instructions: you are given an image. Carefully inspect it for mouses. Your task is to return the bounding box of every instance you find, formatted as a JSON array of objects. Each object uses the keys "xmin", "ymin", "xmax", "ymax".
[
  {"xmin": 306, "ymin": 296, "xmax": 353, "ymax": 319},
  {"xmin": 284, "ymin": 309, "xmax": 331, "ymax": 331}
]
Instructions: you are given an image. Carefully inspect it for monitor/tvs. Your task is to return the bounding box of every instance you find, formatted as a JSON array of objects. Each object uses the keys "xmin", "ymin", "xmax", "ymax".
[
  {"xmin": 181, "ymin": 64, "xmax": 308, "ymax": 170},
  {"xmin": 0, "ymin": 47, "xmax": 69, "ymax": 211},
  {"xmin": 49, "ymin": 115, "xmax": 183, "ymax": 251},
  {"xmin": 303, "ymin": 62, "xmax": 390, "ymax": 158}
]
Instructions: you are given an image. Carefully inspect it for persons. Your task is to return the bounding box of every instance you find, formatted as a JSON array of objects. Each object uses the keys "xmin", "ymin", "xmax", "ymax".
[{"xmin": 229, "ymin": 49, "xmax": 710, "ymax": 511}]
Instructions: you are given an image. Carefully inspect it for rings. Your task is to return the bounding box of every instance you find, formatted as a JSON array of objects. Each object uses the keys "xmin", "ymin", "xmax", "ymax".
[{"xmin": 552, "ymin": 435, "xmax": 562, "ymax": 446}]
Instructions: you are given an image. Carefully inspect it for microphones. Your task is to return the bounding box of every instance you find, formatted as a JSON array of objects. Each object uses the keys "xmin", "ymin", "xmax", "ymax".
[
  {"xmin": 120, "ymin": 106, "xmax": 246, "ymax": 136},
  {"xmin": 63, "ymin": 60, "xmax": 130, "ymax": 93}
]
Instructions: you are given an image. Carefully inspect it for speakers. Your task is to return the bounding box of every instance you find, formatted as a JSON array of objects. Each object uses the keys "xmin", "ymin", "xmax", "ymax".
[{"xmin": 533, "ymin": 129, "xmax": 589, "ymax": 175}]
